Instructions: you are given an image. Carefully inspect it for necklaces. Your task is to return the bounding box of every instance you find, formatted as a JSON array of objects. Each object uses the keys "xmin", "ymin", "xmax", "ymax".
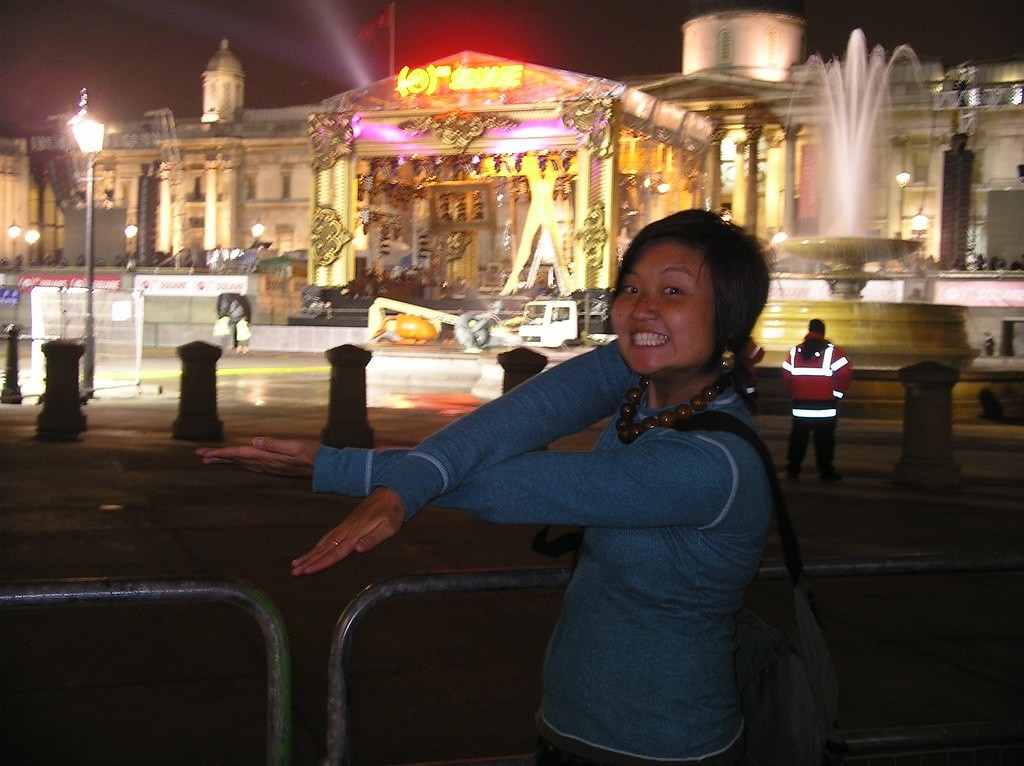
[{"xmin": 614, "ymin": 376, "xmax": 729, "ymax": 443}]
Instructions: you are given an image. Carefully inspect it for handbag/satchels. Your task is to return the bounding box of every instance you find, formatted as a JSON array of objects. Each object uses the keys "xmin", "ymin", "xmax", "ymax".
[{"xmin": 734, "ymin": 583, "xmax": 839, "ymax": 766}]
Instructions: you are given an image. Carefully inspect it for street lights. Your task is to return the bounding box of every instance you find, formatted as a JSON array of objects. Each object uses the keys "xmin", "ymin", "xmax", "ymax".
[
  {"xmin": 896, "ymin": 168, "xmax": 910, "ymax": 240},
  {"xmin": 252, "ymin": 223, "xmax": 265, "ymax": 242},
  {"xmin": 125, "ymin": 225, "xmax": 138, "ymax": 254},
  {"xmin": 9, "ymin": 225, "xmax": 21, "ymax": 325},
  {"xmin": 71, "ymin": 85, "xmax": 104, "ymax": 399},
  {"xmin": 25, "ymin": 230, "xmax": 41, "ymax": 294}
]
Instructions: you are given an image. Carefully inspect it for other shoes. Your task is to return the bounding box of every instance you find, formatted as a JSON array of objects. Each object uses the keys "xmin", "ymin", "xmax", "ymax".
[
  {"xmin": 786, "ymin": 470, "xmax": 798, "ymax": 478},
  {"xmin": 819, "ymin": 470, "xmax": 843, "ymax": 480}
]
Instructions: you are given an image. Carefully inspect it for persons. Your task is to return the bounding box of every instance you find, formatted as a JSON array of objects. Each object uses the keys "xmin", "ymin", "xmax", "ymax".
[
  {"xmin": 780, "ymin": 319, "xmax": 852, "ymax": 484},
  {"xmin": 1010, "ymin": 254, "xmax": 1024, "ymax": 270},
  {"xmin": 983, "ymin": 331, "xmax": 995, "ymax": 356},
  {"xmin": 977, "ymin": 389, "xmax": 1004, "ymax": 418},
  {"xmin": 740, "ymin": 336, "xmax": 764, "ymax": 412},
  {"xmin": 950, "ymin": 254, "xmax": 998, "ymax": 270},
  {"xmin": 212, "ymin": 316, "xmax": 252, "ymax": 355},
  {"xmin": 194, "ymin": 209, "xmax": 779, "ymax": 766}
]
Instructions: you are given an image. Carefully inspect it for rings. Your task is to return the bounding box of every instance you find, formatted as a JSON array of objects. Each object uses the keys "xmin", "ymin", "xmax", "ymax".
[{"xmin": 332, "ymin": 540, "xmax": 339, "ymax": 546}]
